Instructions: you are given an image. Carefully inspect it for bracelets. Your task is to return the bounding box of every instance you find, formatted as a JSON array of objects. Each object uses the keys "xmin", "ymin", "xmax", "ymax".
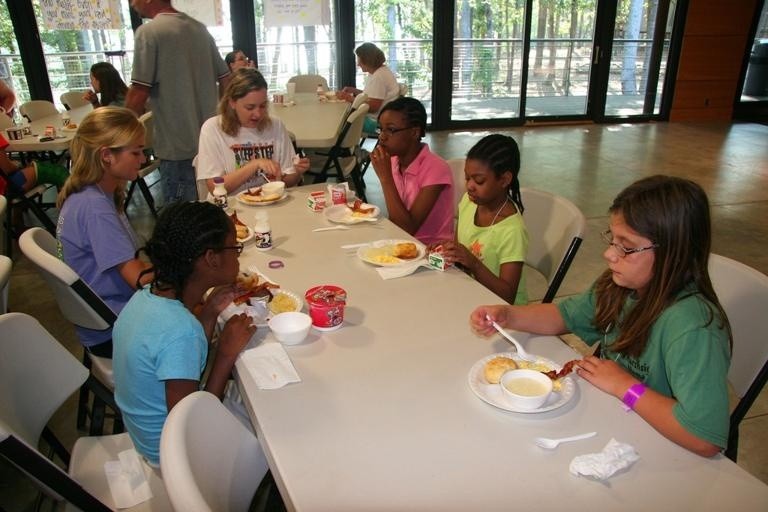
[
  {"xmin": 92, "ymin": 98, "xmax": 98, "ymax": 104},
  {"xmin": 0, "ymin": 107, "xmax": 7, "ymax": 113},
  {"xmin": 620, "ymin": 383, "xmax": 647, "ymax": 408}
]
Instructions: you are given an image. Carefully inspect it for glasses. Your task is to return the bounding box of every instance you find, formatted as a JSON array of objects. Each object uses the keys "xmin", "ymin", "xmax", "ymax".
[
  {"xmin": 599, "ymin": 229, "xmax": 661, "ymax": 259},
  {"xmin": 221, "ymin": 241, "xmax": 244, "ymax": 254},
  {"xmin": 374, "ymin": 125, "xmax": 416, "ymax": 137}
]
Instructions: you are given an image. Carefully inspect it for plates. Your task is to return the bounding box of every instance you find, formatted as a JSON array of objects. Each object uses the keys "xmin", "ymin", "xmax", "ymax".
[
  {"xmin": 63, "ymin": 124, "xmax": 80, "ymax": 132},
  {"xmin": 323, "ymin": 201, "xmax": 381, "ymax": 224},
  {"xmin": 228, "ymin": 287, "xmax": 303, "ymax": 325},
  {"xmin": 235, "ymin": 186, "xmax": 292, "ymax": 208},
  {"xmin": 356, "ymin": 237, "xmax": 426, "ymax": 266},
  {"xmin": 467, "ymin": 352, "xmax": 576, "ymax": 412},
  {"xmin": 231, "ymin": 218, "xmax": 252, "ymax": 246}
]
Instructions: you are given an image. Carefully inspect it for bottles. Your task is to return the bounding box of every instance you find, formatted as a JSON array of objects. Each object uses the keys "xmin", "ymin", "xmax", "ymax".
[
  {"xmin": 253, "ymin": 209, "xmax": 273, "ymax": 252},
  {"xmin": 310, "ymin": 191, "xmax": 326, "ymax": 212},
  {"xmin": 316, "ymin": 82, "xmax": 324, "ymax": 98},
  {"xmin": 212, "ymin": 175, "xmax": 230, "ymax": 210}
]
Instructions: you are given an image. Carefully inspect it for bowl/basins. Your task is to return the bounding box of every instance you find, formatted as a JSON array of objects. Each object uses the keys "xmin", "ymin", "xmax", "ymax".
[
  {"xmin": 502, "ymin": 368, "xmax": 553, "ymax": 409},
  {"xmin": 261, "ymin": 180, "xmax": 287, "ymax": 198},
  {"xmin": 267, "ymin": 310, "xmax": 313, "ymax": 346}
]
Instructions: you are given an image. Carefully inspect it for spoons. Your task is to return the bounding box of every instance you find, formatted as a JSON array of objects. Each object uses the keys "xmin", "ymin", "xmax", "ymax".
[
  {"xmin": 248, "ymin": 264, "xmax": 279, "ymax": 288},
  {"xmin": 484, "ymin": 315, "xmax": 541, "ymax": 366},
  {"xmin": 343, "ymin": 243, "xmax": 367, "ymax": 252},
  {"xmin": 531, "ymin": 430, "xmax": 600, "ymax": 451},
  {"xmin": 345, "ymin": 214, "xmax": 376, "ymax": 222},
  {"xmin": 311, "ymin": 224, "xmax": 349, "ymax": 234}
]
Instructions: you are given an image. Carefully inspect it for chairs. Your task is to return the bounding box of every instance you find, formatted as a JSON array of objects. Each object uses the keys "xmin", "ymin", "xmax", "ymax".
[
  {"xmin": 17, "ymin": 226, "xmax": 126, "ymax": 438},
  {"xmin": 510, "ymin": 185, "xmax": 586, "ymax": 309},
  {"xmin": 155, "ymin": 388, "xmax": 267, "ymax": 512},
  {"xmin": 1, "ymin": 71, "xmax": 406, "ymax": 224},
  {"xmin": 694, "ymin": 251, "xmax": 767, "ymax": 471},
  {"xmin": 445, "ymin": 156, "xmax": 481, "ymax": 241},
  {"xmin": 0, "ymin": 310, "xmax": 174, "ymax": 512}
]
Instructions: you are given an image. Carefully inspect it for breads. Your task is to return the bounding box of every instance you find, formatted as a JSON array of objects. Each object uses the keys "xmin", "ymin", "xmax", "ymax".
[
  {"xmin": 239, "ymin": 194, "xmax": 280, "ymax": 203},
  {"xmin": 235, "ymin": 224, "xmax": 248, "ymax": 239},
  {"xmin": 353, "ymin": 206, "xmax": 373, "ymax": 215},
  {"xmin": 237, "ymin": 271, "xmax": 258, "ymax": 292},
  {"xmin": 484, "ymin": 356, "xmax": 518, "ymax": 383},
  {"xmin": 392, "ymin": 242, "xmax": 419, "ymax": 259}
]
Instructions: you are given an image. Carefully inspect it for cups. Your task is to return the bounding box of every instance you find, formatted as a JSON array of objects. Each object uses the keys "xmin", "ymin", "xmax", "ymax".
[
  {"xmin": 6, "ymin": 126, "xmax": 30, "ymax": 140},
  {"xmin": 287, "ymin": 83, "xmax": 297, "ymax": 96}
]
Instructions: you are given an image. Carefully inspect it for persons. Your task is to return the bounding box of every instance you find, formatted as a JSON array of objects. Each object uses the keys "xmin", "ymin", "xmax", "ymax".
[
  {"xmin": 80, "ymin": 61, "xmax": 151, "ymax": 169},
  {"xmin": 0, "ymin": 71, "xmax": 17, "ymax": 116},
  {"xmin": 111, "ymin": 199, "xmax": 282, "ymax": 508},
  {"xmin": 54, "ymin": 104, "xmax": 160, "ymax": 359},
  {"xmin": 197, "ymin": 67, "xmax": 311, "ymax": 199},
  {"xmin": 469, "ymin": 173, "xmax": 733, "ymax": 460},
  {"xmin": 333, "ymin": 40, "xmax": 400, "ymax": 183},
  {"xmin": 120, "ymin": 0, "xmax": 234, "ymax": 219},
  {"xmin": 0, "ymin": 130, "xmax": 70, "ymax": 200},
  {"xmin": 424, "ymin": 132, "xmax": 528, "ymax": 306},
  {"xmin": 225, "ymin": 49, "xmax": 256, "ymax": 73},
  {"xmin": 368, "ymin": 96, "xmax": 456, "ymax": 252}
]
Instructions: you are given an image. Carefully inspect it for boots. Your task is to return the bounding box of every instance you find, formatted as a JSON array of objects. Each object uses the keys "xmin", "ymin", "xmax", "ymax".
[{"xmin": 29, "ymin": 160, "xmax": 71, "ymax": 190}]
